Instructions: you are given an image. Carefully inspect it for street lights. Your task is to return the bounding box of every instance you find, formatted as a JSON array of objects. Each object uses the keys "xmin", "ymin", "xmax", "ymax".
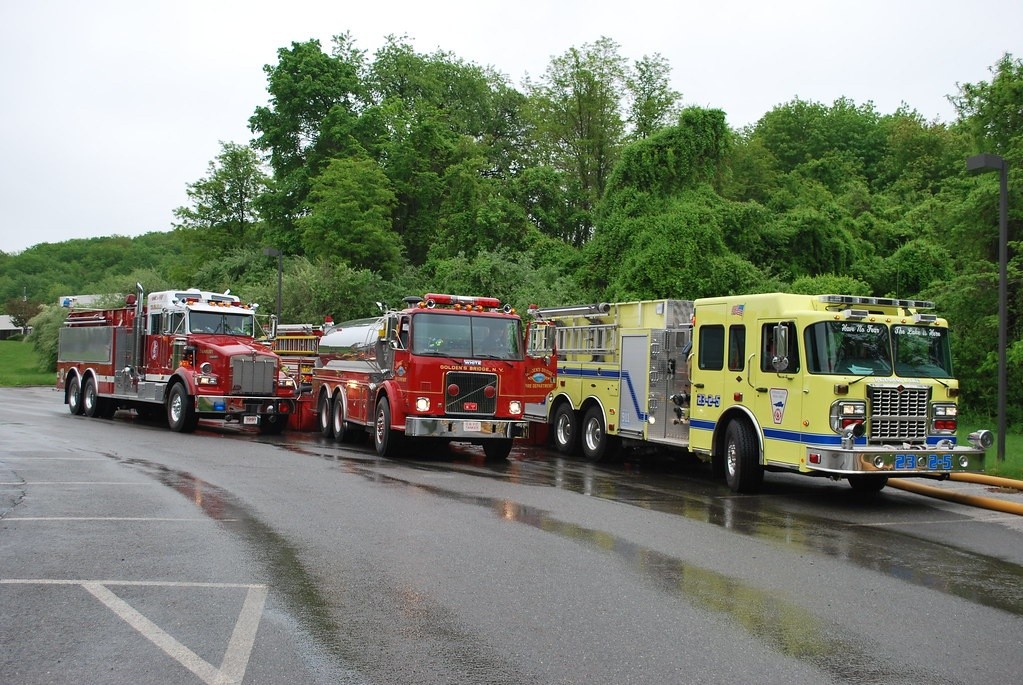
[
  {"xmin": 966, "ymin": 155, "xmax": 1010, "ymax": 461},
  {"xmin": 262, "ymin": 245, "xmax": 283, "ymax": 324}
]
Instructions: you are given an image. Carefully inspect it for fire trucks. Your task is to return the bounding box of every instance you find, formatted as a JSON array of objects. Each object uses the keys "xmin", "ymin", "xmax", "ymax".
[
  {"xmin": 524, "ymin": 291, "xmax": 993, "ymax": 494},
  {"xmin": 56, "ymin": 280, "xmax": 301, "ymax": 435},
  {"xmin": 304, "ymin": 290, "xmax": 530, "ymax": 463}
]
